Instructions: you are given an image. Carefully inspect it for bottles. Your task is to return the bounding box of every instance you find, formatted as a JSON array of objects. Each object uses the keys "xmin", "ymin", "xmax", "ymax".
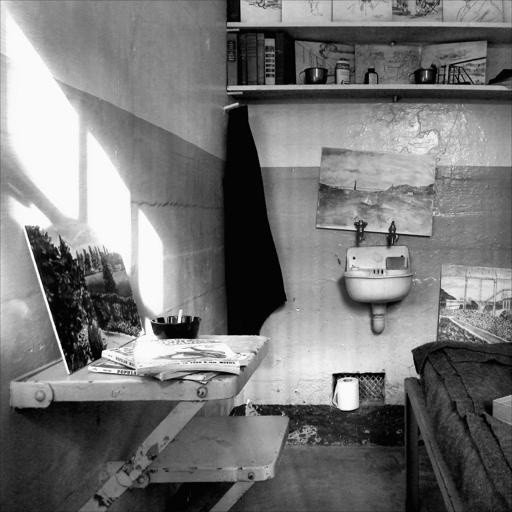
[
  {"xmin": 334, "ymin": 61, "xmax": 351, "ymax": 84},
  {"xmin": 364, "ymin": 66, "xmax": 379, "ymax": 84}
]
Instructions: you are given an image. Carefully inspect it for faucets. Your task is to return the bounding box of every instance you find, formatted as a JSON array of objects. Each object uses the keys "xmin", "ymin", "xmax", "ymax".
[
  {"xmin": 354, "ymin": 220, "xmax": 367, "ymax": 242},
  {"xmin": 386, "ymin": 221, "xmax": 400, "ymax": 246}
]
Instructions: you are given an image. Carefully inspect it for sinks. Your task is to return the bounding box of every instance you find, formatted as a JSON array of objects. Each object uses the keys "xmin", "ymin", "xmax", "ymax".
[{"xmin": 344, "ymin": 246, "xmax": 414, "ymax": 304}]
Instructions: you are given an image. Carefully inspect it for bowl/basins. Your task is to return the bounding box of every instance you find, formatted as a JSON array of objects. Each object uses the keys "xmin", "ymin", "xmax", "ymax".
[{"xmin": 151, "ymin": 315, "xmax": 202, "ymax": 340}]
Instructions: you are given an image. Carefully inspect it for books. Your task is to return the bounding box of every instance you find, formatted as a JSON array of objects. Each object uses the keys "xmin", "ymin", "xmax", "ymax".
[
  {"xmin": 225, "ymin": 31, "xmax": 295, "ymax": 87},
  {"xmin": 86, "ymin": 337, "xmax": 257, "ymax": 386}
]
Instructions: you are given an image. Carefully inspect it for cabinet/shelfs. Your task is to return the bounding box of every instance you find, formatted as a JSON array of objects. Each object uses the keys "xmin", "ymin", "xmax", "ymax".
[
  {"xmin": 8, "ymin": 333, "xmax": 290, "ymax": 512},
  {"xmin": 227, "ymin": 21, "xmax": 512, "ymax": 99}
]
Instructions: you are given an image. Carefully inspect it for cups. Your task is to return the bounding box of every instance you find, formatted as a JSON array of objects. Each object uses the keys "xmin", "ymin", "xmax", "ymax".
[
  {"xmin": 409, "ymin": 69, "xmax": 436, "ymax": 84},
  {"xmin": 299, "ymin": 67, "xmax": 328, "ymax": 84}
]
